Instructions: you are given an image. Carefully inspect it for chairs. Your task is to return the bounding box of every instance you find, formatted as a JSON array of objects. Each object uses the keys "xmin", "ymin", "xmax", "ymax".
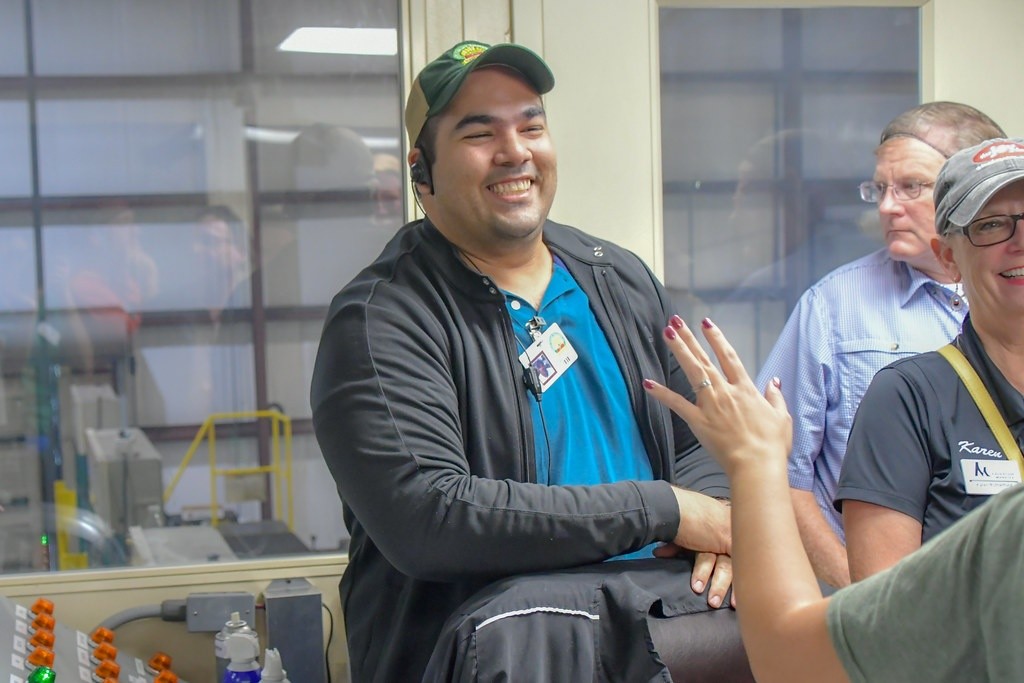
[{"xmin": 422, "ymin": 558, "xmax": 756, "ymax": 683}]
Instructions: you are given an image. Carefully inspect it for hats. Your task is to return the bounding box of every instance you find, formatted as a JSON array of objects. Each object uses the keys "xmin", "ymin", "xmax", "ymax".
[
  {"xmin": 405, "ymin": 40, "xmax": 555, "ymax": 151},
  {"xmin": 934, "ymin": 138, "xmax": 1024, "ymax": 236},
  {"xmin": 880, "ymin": 101, "xmax": 1008, "ymax": 160}
]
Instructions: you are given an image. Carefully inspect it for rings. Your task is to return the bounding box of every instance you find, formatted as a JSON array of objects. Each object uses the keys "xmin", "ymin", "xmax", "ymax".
[{"xmin": 693, "ymin": 380, "xmax": 711, "ymax": 391}]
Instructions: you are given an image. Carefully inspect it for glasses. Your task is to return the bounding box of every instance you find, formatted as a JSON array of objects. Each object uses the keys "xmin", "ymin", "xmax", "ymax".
[
  {"xmin": 940, "ymin": 213, "xmax": 1024, "ymax": 247},
  {"xmin": 858, "ymin": 179, "xmax": 935, "ymax": 203}
]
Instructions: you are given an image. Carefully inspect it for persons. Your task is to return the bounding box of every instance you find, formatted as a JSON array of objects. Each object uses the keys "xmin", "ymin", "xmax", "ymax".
[
  {"xmin": 310, "ymin": 39, "xmax": 838, "ymax": 683},
  {"xmin": 641, "ymin": 314, "xmax": 1024, "ymax": 682},
  {"xmin": 38, "ymin": 120, "xmax": 401, "ymax": 547},
  {"xmin": 713, "ymin": 129, "xmax": 883, "ymax": 371},
  {"xmin": 759, "ymin": 102, "xmax": 1007, "ymax": 589},
  {"xmin": 536, "ymin": 360, "xmax": 554, "ymax": 382},
  {"xmin": 834, "ymin": 137, "xmax": 1024, "ymax": 582}
]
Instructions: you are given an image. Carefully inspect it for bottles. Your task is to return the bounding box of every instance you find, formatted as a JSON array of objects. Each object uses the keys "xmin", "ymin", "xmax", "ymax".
[
  {"xmin": 222, "ymin": 633, "xmax": 263, "ymax": 683},
  {"xmin": 258, "ymin": 648, "xmax": 291, "ymax": 683},
  {"xmin": 142, "ymin": 505, "xmax": 166, "ymax": 531},
  {"xmin": 214, "ymin": 612, "xmax": 259, "ymax": 683}
]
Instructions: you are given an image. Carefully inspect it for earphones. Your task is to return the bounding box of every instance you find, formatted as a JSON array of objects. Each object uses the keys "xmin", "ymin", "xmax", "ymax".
[{"xmin": 409, "ymin": 150, "xmax": 433, "ymax": 196}]
